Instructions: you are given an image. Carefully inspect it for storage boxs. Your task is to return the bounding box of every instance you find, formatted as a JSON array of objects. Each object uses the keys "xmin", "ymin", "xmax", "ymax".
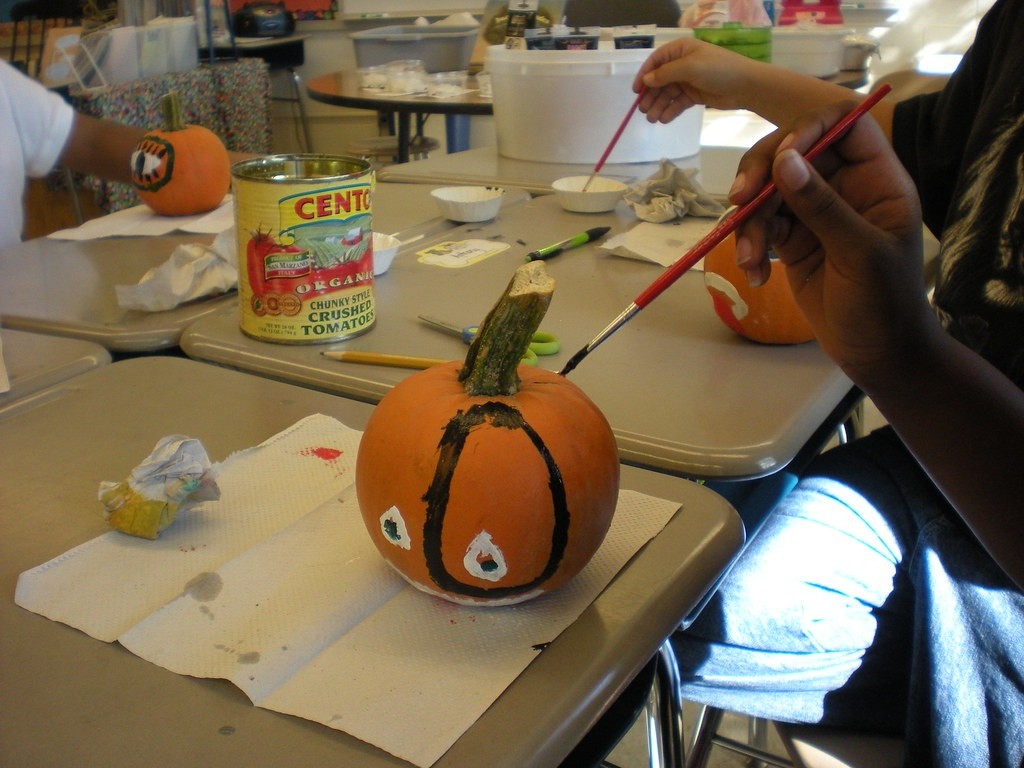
[
  {"xmin": 613, "ymin": 26, "xmax": 854, "ymax": 78},
  {"xmin": 346, "ymin": 24, "xmax": 479, "ymax": 73}
]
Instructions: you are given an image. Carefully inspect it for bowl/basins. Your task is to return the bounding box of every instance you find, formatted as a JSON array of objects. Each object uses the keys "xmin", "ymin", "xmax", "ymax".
[
  {"xmin": 359, "ymin": 59, "xmax": 468, "ymax": 97},
  {"xmin": 552, "ymin": 175, "xmax": 628, "ymax": 213},
  {"xmin": 431, "ymin": 185, "xmax": 506, "ymax": 223},
  {"xmin": 771, "ymin": 26, "xmax": 855, "ymax": 78},
  {"xmin": 840, "ymin": 41, "xmax": 879, "ymax": 72},
  {"xmin": 484, "ymin": 41, "xmax": 704, "ymax": 165},
  {"xmin": 691, "ymin": 21, "xmax": 773, "ymax": 65},
  {"xmin": 372, "ymin": 231, "xmax": 402, "ymax": 276}
]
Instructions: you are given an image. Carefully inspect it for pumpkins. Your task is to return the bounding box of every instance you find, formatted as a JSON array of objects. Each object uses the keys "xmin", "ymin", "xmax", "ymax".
[
  {"xmin": 703, "ymin": 202, "xmax": 813, "ymax": 343},
  {"xmin": 356, "ymin": 262, "xmax": 620, "ymax": 605},
  {"xmin": 132, "ymin": 81, "xmax": 229, "ymax": 215}
]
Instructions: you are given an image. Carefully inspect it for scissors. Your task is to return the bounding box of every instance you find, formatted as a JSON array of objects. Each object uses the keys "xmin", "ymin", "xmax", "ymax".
[{"xmin": 417, "ymin": 313, "xmax": 561, "ymax": 368}]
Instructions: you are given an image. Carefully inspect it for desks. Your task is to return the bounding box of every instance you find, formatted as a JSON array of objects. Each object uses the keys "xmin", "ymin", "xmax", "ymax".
[
  {"xmin": 305, "ymin": 63, "xmax": 873, "ymax": 165},
  {"xmin": 0, "ymin": 328, "xmax": 112, "ymax": 406},
  {"xmin": 0, "ymin": 180, "xmax": 531, "ymax": 352},
  {"xmin": 0, "ymin": 355, "xmax": 747, "ymax": 768},
  {"xmin": 178, "ymin": 192, "xmax": 868, "ymax": 768},
  {"xmin": 376, "ymin": 147, "xmax": 752, "ymax": 208}
]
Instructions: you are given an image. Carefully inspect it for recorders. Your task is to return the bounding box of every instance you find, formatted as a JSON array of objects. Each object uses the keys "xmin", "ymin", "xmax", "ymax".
[{"xmin": 232, "ymin": 0, "xmax": 296, "ymax": 38}]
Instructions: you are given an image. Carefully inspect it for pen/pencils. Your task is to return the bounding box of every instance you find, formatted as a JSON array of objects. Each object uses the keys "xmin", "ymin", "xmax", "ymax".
[
  {"xmin": 524, "ymin": 226, "xmax": 612, "ymax": 262},
  {"xmin": 318, "ymin": 350, "xmax": 450, "ymax": 370}
]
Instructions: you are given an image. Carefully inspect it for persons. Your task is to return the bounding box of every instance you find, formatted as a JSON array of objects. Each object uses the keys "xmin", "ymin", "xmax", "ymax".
[
  {"xmin": 727, "ymin": 100, "xmax": 1024, "ymax": 590},
  {"xmin": 628, "ymin": 0, "xmax": 1023, "ymax": 768},
  {"xmin": 0, "ymin": 57, "xmax": 270, "ymax": 253}
]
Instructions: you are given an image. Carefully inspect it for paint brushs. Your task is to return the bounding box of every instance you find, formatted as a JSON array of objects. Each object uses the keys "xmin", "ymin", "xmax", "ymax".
[
  {"xmin": 583, "ymin": 83, "xmax": 650, "ymax": 192},
  {"xmin": 555, "ymin": 82, "xmax": 896, "ymax": 378}
]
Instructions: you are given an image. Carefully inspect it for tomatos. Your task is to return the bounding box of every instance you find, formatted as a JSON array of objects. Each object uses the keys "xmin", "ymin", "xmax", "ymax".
[{"xmin": 247, "ymin": 226, "xmax": 373, "ymax": 301}]
